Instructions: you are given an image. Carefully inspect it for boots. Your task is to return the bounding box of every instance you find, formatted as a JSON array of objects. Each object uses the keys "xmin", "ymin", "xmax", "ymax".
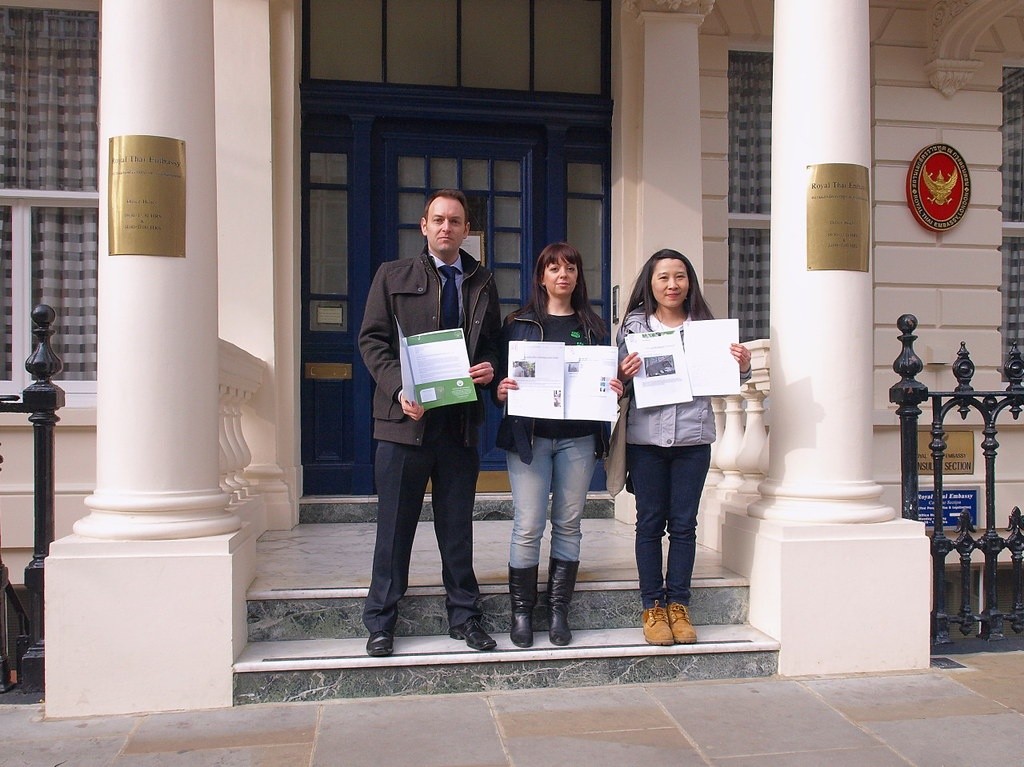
[
  {"xmin": 545, "ymin": 556, "xmax": 581, "ymax": 646},
  {"xmin": 505, "ymin": 561, "xmax": 540, "ymax": 649}
]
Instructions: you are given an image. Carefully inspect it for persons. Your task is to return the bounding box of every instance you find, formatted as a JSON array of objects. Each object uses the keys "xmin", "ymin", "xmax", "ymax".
[
  {"xmin": 358, "ymin": 189, "xmax": 501, "ymax": 657},
  {"xmin": 615, "ymin": 249, "xmax": 752, "ymax": 646},
  {"xmin": 489, "ymin": 240, "xmax": 623, "ymax": 648}
]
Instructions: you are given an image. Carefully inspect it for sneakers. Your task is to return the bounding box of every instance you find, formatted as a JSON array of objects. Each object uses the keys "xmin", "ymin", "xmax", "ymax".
[
  {"xmin": 665, "ymin": 601, "xmax": 698, "ymax": 644},
  {"xmin": 642, "ymin": 604, "xmax": 675, "ymax": 646}
]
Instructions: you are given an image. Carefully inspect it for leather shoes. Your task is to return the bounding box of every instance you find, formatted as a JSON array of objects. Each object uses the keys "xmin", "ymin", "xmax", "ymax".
[
  {"xmin": 364, "ymin": 631, "xmax": 396, "ymax": 656},
  {"xmin": 449, "ymin": 616, "xmax": 498, "ymax": 651}
]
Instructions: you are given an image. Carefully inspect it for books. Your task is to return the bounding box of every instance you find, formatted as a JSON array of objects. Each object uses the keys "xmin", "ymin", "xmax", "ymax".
[
  {"xmin": 624, "ymin": 318, "xmax": 741, "ymax": 410},
  {"xmin": 508, "ymin": 340, "xmax": 619, "ymax": 422},
  {"xmin": 394, "ymin": 315, "xmax": 479, "ymax": 411}
]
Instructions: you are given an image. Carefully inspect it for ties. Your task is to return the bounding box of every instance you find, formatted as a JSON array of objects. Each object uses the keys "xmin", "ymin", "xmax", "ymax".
[{"xmin": 436, "ymin": 264, "xmax": 461, "ymax": 332}]
